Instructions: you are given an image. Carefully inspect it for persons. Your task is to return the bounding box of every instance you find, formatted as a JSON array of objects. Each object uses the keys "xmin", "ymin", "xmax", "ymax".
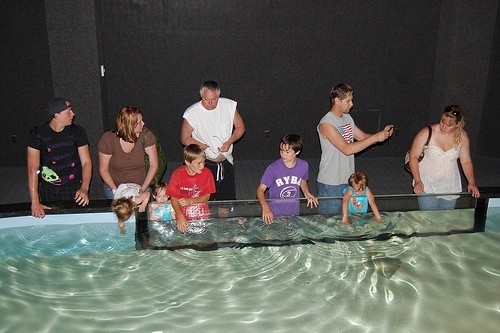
[
  {"xmin": 180, "ymin": 81, "xmax": 245, "ymax": 217},
  {"xmin": 341, "ymin": 172, "xmax": 382, "ymax": 224},
  {"xmin": 316, "ymin": 83, "xmax": 394, "ymax": 215},
  {"xmin": 147, "ymin": 181, "xmax": 176, "ymax": 221},
  {"xmin": 98, "ymin": 105, "xmax": 159, "ymax": 199},
  {"xmin": 166, "ymin": 144, "xmax": 216, "ymax": 231},
  {"xmin": 111, "ymin": 182, "xmax": 151, "ymax": 234},
  {"xmin": 409, "ymin": 105, "xmax": 480, "ymax": 210},
  {"xmin": 27, "ymin": 98, "xmax": 93, "ymax": 218},
  {"xmin": 256, "ymin": 133, "xmax": 319, "ymax": 224}
]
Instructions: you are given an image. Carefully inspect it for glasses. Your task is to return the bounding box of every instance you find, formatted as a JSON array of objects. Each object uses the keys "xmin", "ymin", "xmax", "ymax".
[{"xmin": 444, "ymin": 106, "xmax": 459, "ymax": 117}]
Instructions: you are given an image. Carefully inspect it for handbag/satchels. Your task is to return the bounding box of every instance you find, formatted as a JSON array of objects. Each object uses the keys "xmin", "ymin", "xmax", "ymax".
[
  {"xmin": 143, "ymin": 131, "xmax": 167, "ymax": 185},
  {"xmin": 404, "ymin": 126, "xmax": 432, "ymax": 172}
]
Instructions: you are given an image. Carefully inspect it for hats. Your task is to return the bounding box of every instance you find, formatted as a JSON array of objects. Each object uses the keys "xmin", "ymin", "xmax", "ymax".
[{"xmin": 48, "ymin": 98, "xmax": 71, "ymax": 116}]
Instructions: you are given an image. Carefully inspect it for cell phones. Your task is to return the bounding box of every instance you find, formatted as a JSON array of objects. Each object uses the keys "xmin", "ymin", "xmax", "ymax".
[{"xmin": 388, "ymin": 127, "xmax": 393, "ymax": 132}]
